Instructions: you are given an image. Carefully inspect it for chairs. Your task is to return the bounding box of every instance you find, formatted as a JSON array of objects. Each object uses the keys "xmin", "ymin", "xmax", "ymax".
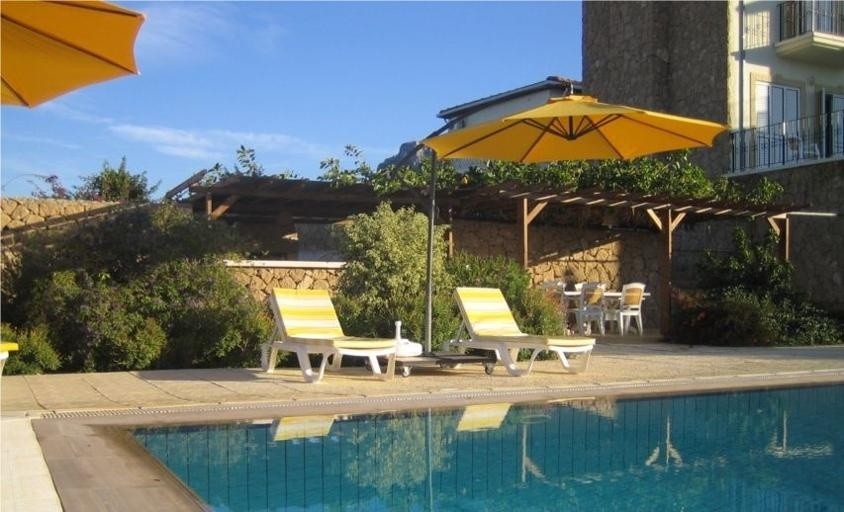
[
  {"xmin": 440, "ymin": 286, "xmax": 597, "ymax": 385},
  {"xmin": 261, "ymin": 285, "xmax": 411, "ymax": 385},
  {"xmin": 541, "ymin": 281, "xmax": 646, "ymax": 337}
]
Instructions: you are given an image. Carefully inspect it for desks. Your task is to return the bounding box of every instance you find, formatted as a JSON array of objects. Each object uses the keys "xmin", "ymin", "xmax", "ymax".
[{"xmin": 561, "ymin": 291, "xmax": 651, "ymax": 336}]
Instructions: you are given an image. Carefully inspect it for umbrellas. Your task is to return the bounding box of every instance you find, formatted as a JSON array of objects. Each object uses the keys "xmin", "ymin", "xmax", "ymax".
[
  {"xmin": 0, "ymin": 1, "xmax": 145, "ymax": 108},
  {"xmin": 388, "ymin": 80, "xmax": 733, "ymax": 352}
]
